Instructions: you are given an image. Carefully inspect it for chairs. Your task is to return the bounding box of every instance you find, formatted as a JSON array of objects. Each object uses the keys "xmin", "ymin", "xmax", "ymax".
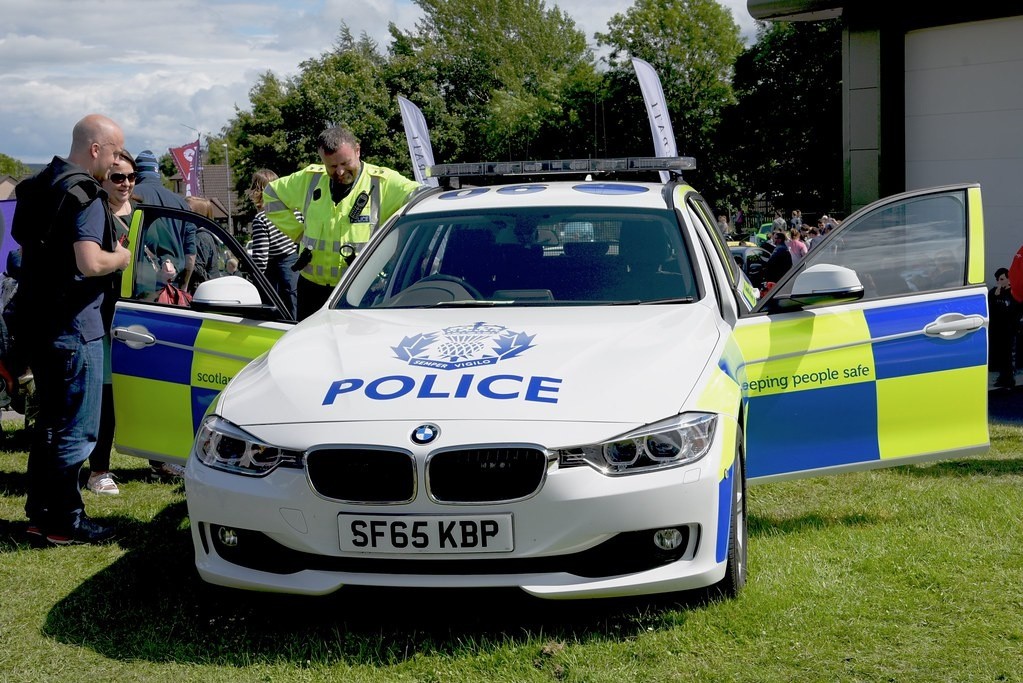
[
  {"xmin": 454, "ymin": 217, "xmax": 546, "ymax": 301},
  {"xmin": 592, "ymin": 221, "xmax": 688, "ymax": 304}
]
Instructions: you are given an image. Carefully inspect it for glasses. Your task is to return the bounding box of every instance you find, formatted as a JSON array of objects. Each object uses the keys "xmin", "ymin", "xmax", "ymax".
[{"xmin": 108, "ymin": 171, "xmax": 137, "ymax": 183}]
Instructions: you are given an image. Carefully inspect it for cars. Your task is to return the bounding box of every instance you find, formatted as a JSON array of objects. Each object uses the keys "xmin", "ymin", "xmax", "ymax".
[
  {"xmin": 106, "ymin": 155, "xmax": 992, "ymax": 611},
  {"xmin": 755, "ymin": 221, "xmax": 791, "ymax": 247},
  {"xmin": 727, "ymin": 244, "xmax": 775, "ymax": 288}
]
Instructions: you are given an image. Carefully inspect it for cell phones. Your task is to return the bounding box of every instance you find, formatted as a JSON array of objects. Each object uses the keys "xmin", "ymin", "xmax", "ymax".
[{"xmin": 119, "ymin": 234, "xmax": 130, "ymax": 250}]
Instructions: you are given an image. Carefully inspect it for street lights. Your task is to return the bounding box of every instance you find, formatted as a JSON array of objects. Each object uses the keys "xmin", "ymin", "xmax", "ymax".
[{"xmin": 182, "ymin": 121, "xmax": 232, "ymax": 237}]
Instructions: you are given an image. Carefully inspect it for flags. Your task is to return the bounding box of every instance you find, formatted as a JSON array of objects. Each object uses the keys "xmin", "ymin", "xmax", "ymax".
[
  {"xmin": 168, "ymin": 140, "xmax": 200, "ymax": 197},
  {"xmin": 397, "ymin": 95, "xmax": 439, "ymax": 189},
  {"xmin": 631, "ymin": 58, "xmax": 684, "ymax": 183}
]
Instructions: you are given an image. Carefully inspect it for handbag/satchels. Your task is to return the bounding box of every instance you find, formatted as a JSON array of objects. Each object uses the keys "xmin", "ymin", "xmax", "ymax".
[{"xmin": 154, "ymin": 281, "xmax": 192, "ymax": 309}]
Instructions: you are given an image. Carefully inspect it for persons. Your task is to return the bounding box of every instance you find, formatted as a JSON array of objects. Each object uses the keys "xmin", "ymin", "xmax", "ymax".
[
  {"xmin": 988, "ymin": 267, "xmax": 1021, "ymax": 389},
  {"xmin": 8, "ymin": 113, "xmax": 133, "ymax": 545},
  {"xmin": 261, "ymin": 124, "xmax": 426, "ymax": 323},
  {"xmin": 932, "ymin": 250, "xmax": 961, "ymax": 288},
  {"xmin": 132, "ymin": 150, "xmax": 196, "ymax": 295},
  {"xmin": 718, "ymin": 215, "xmax": 730, "ymax": 234},
  {"xmin": 733, "ymin": 206, "xmax": 745, "ymax": 234},
  {"xmin": 225, "ymin": 259, "xmax": 242, "ymax": 276},
  {"xmin": 185, "ymin": 197, "xmax": 223, "ymax": 297},
  {"xmin": 223, "ymin": 250, "xmax": 234, "ymax": 267},
  {"xmin": 248, "ymin": 168, "xmax": 299, "ymax": 323},
  {"xmin": 761, "ymin": 210, "xmax": 845, "ymax": 283},
  {"xmin": 86, "ymin": 148, "xmax": 187, "ymax": 495}
]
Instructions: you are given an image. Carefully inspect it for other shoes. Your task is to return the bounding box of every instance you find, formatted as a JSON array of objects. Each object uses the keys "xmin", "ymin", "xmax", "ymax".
[{"xmin": 993, "ymin": 374, "xmax": 1017, "ymax": 389}]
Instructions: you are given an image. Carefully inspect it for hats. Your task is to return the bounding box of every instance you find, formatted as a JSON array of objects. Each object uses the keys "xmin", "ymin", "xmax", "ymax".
[{"xmin": 134, "ymin": 150, "xmax": 159, "ymax": 173}]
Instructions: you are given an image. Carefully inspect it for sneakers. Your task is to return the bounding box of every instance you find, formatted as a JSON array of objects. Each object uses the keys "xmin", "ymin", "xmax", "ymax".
[
  {"xmin": 151, "ymin": 462, "xmax": 186, "ymax": 483},
  {"xmin": 87, "ymin": 471, "xmax": 120, "ymax": 495},
  {"xmin": 27, "ymin": 512, "xmax": 118, "ymax": 545}
]
